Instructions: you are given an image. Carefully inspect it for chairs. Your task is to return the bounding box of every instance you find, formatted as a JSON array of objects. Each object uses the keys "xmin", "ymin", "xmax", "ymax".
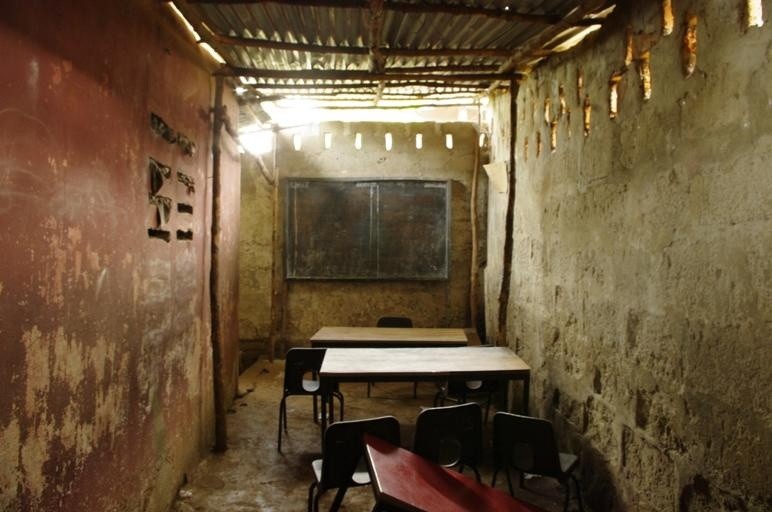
[
  {"xmin": 365, "ymin": 315, "xmax": 418, "ymax": 404},
  {"xmin": 302, "ymin": 412, "xmax": 401, "ymax": 511},
  {"xmin": 409, "ymin": 402, "xmax": 487, "ymax": 487},
  {"xmin": 279, "ymin": 348, "xmax": 332, "ymax": 451},
  {"xmin": 491, "ymin": 411, "xmax": 587, "ymax": 510}
]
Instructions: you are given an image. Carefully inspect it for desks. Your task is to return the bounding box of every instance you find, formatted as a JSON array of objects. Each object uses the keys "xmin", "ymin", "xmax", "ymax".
[
  {"xmin": 331, "ymin": 433, "xmax": 540, "ymax": 510},
  {"xmin": 311, "ymin": 324, "xmax": 470, "ymax": 427},
  {"xmin": 320, "ymin": 346, "xmax": 531, "ymax": 434}
]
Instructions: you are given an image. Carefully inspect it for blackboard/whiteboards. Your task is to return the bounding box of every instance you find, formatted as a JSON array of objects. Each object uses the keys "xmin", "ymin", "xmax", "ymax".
[{"xmin": 280, "ymin": 177, "xmax": 451, "ymax": 282}]
